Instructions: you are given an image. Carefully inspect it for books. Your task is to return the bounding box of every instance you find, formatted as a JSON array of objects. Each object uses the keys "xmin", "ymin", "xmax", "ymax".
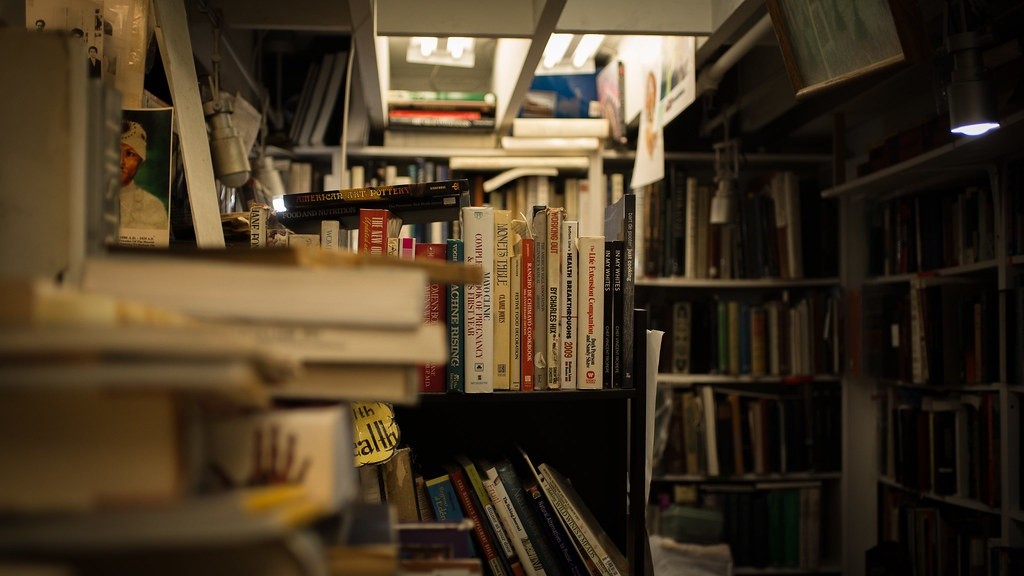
[{"xmin": 0, "ymin": 0, "xmax": 1024, "ymax": 576}]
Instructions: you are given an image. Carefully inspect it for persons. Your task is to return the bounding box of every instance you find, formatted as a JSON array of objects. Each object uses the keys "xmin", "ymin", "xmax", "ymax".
[
  {"xmin": 118, "ymin": 119, "xmax": 168, "ymax": 230},
  {"xmin": 88, "ymin": 46, "xmax": 101, "ymax": 78},
  {"xmin": 71, "ymin": 28, "xmax": 84, "ymax": 45},
  {"xmin": 35, "ymin": 20, "xmax": 45, "ymax": 32},
  {"xmin": 95, "ymin": 15, "xmax": 102, "ymax": 31}
]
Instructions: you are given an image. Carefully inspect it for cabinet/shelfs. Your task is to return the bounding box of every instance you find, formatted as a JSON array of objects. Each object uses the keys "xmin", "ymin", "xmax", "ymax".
[{"xmin": 0, "ymin": 0, "xmax": 1024, "ymax": 575}]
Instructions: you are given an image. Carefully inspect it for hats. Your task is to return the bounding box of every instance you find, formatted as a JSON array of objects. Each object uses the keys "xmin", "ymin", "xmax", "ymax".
[{"xmin": 121, "ymin": 120, "xmax": 146, "ymax": 161}]
